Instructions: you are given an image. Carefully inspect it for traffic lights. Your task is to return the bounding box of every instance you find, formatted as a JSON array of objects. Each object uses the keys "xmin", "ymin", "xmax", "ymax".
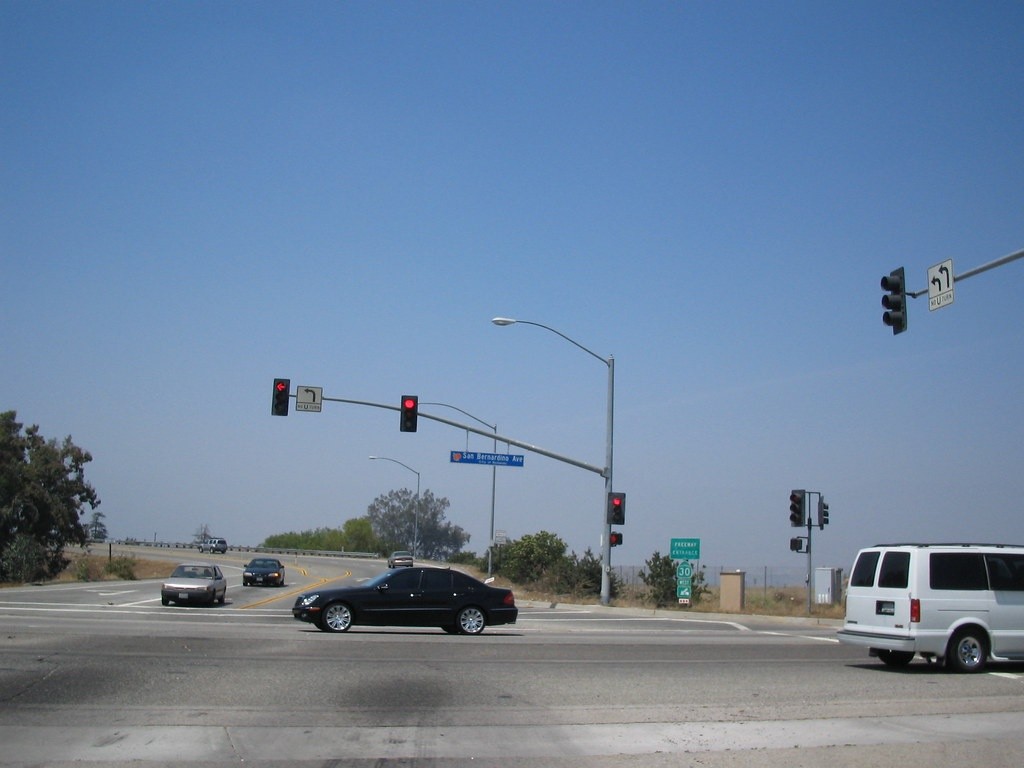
[
  {"xmin": 790, "ymin": 538, "xmax": 802, "ymax": 550},
  {"xmin": 880, "ymin": 265, "xmax": 908, "ymax": 335},
  {"xmin": 790, "ymin": 489, "xmax": 805, "ymax": 527},
  {"xmin": 611, "ymin": 534, "xmax": 622, "ymax": 547},
  {"xmin": 607, "ymin": 492, "xmax": 626, "ymax": 525},
  {"xmin": 818, "ymin": 496, "xmax": 829, "ymax": 525},
  {"xmin": 271, "ymin": 378, "xmax": 291, "ymax": 416},
  {"xmin": 400, "ymin": 395, "xmax": 419, "ymax": 432}
]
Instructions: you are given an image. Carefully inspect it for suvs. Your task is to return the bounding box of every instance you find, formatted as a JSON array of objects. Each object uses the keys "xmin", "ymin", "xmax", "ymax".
[
  {"xmin": 834, "ymin": 541, "xmax": 1024, "ymax": 675},
  {"xmin": 197, "ymin": 537, "xmax": 228, "ymax": 554}
]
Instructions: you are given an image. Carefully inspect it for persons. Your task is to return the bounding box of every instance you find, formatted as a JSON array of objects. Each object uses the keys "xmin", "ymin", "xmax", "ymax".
[
  {"xmin": 203, "ymin": 569, "xmax": 212, "ymax": 576},
  {"xmin": 173, "ymin": 566, "xmax": 189, "ymax": 577},
  {"xmin": 270, "ymin": 563, "xmax": 277, "ymax": 568}
]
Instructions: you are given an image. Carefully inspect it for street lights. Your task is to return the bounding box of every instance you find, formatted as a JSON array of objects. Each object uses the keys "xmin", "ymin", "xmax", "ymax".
[
  {"xmin": 490, "ymin": 317, "xmax": 616, "ymax": 605},
  {"xmin": 368, "ymin": 455, "xmax": 421, "ymax": 567}
]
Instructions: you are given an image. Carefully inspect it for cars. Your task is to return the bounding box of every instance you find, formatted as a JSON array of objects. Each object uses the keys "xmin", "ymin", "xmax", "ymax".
[
  {"xmin": 161, "ymin": 563, "xmax": 227, "ymax": 608},
  {"xmin": 242, "ymin": 557, "xmax": 285, "ymax": 587},
  {"xmin": 388, "ymin": 551, "xmax": 414, "ymax": 569},
  {"xmin": 291, "ymin": 567, "xmax": 520, "ymax": 635}
]
